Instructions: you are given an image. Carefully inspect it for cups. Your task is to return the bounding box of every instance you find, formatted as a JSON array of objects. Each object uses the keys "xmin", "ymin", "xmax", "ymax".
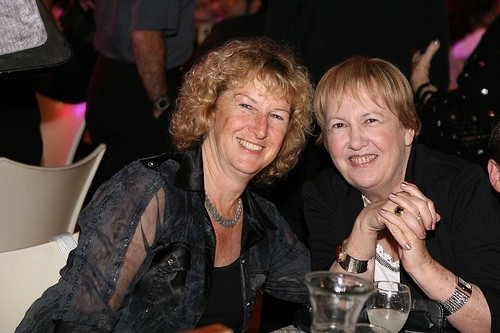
[
  {"xmin": 304, "ymin": 272, "xmax": 373, "ymax": 333},
  {"xmin": 367, "ymin": 281, "xmax": 411, "ymax": 333}
]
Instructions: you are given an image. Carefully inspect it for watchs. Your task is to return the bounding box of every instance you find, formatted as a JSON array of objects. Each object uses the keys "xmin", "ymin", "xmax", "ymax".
[
  {"xmin": 152, "ymin": 94, "xmax": 173, "ymax": 111},
  {"xmin": 441, "ymin": 274, "xmax": 472, "ymax": 317}
]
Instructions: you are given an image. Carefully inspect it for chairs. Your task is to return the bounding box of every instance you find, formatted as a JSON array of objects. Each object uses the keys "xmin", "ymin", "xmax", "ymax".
[
  {"xmin": 0, "ymin": 144, "xmax": 106, "ymax": 253},
  {"xmin": 35, "ymin": 92, "xmax": 86, "ymax": 167},
  {"xmin": 0, "ymin": 231, "xmax": 80, "ymax": 333}
]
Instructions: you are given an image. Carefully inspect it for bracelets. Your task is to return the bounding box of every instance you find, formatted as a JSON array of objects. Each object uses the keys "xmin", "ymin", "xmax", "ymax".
[
  {"xmin": 335, "ymin": 239, "xmax": 376, "ymax": 274},
  {"xmin": 415, "ymin": 81, "xmax": 438, "ymax": 106}
]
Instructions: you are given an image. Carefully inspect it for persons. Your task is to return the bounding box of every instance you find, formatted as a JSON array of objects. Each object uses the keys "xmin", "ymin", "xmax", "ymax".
[
  {"xmin": 0, "ymin": 1, "xmax": 98, "ymax": 168},
  {"xmin": 14, "ymin": 37, "xmax": 315, "ymax": 332},
  {"xmin": 409, "ymin": 13, "xmax": 500, "ymax": 165},
  {"xmin": 196, "ymin": 0, "xmax": 267, "ymax": 54},
  {"xmin": 85, "ymin": 0, "xmax": 195, "ymax": 185},
  {"xmin": 484, "ymin": 125, "xmax": 500, "ymax": 217},
  {"xmin": 303, "ymin": 57, "xmax": 500, "ymax": 333}
]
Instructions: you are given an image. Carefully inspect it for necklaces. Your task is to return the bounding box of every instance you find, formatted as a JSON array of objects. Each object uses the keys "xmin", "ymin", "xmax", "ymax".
[{"xmin": 205, "ymin": 190, "xmax": 243, "ymax": 229}]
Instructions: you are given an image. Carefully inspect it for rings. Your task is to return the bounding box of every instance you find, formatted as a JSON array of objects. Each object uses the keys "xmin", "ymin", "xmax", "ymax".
[
  {"xmin": 415, "ymin": 212, "xmax": 422, "ymax": 221},
  {"xmin": 394, "ymin": 206, "xmax": 405, "ymax": 216}
]
